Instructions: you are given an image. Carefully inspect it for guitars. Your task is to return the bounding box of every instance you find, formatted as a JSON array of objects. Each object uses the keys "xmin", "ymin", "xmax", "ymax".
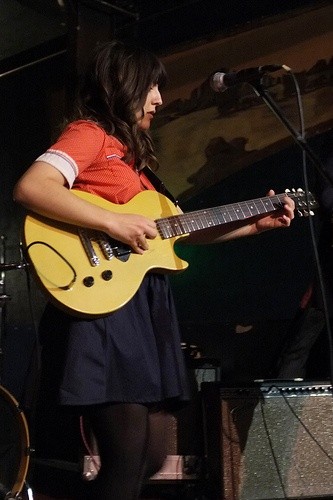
[{"xmin": 22, "ymin": 187, "xmax": 319, "ymax": 320}]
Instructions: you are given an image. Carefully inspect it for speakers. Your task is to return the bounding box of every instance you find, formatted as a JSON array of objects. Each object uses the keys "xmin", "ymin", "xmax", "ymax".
[
  {"xmin": 177, "ymin": 354, "xmax": 225, "ymax": 460},
  {"xmin": 200, "ymin": 381, "xmax": 333, "ymax": 500}
]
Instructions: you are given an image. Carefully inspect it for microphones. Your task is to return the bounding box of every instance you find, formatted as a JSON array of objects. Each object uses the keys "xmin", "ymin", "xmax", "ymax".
[{"xmin": 211, "ymin": 63, "xmax": 285, "ymax": 92}]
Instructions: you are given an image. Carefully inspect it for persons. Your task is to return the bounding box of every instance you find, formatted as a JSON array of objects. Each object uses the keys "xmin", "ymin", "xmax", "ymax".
[{"xmin": 13, "ymin": 40, "xmax": 296, "ymax": 500}]
